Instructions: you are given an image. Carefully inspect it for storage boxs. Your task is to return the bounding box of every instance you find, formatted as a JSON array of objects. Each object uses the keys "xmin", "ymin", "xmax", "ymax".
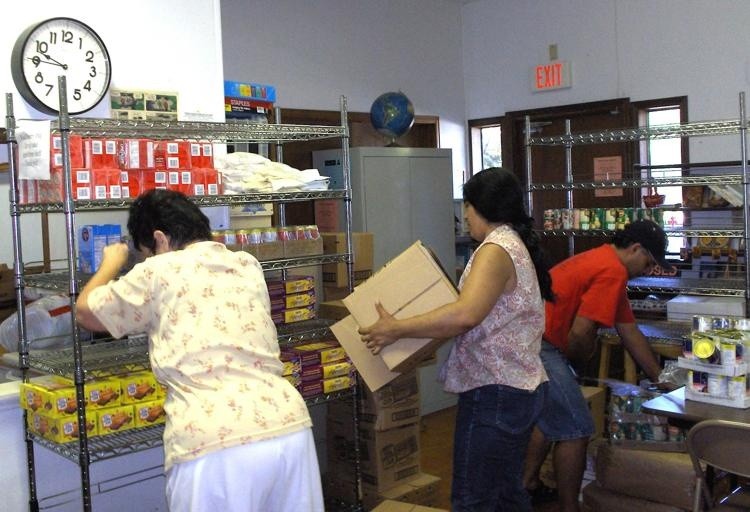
[
  {"xmin": 577, "ymin": 383, "xmax": 606, "ymax": 443},
  {"xmin": 582, "ymin": 442, "xmax": 709, "ymax": 512},
  {"xmin": 318, "ymin": 227, "xmax": 458, "ymax": 512},
  {"xmin": 665, "ymin": 292, "xmax": 747, "ymax": 326}
]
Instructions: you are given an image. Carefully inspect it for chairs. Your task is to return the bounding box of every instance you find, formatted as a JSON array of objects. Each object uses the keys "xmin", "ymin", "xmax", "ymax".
[{"xmin": 687, "ymin": 417, "xmax": 750, "ymax": 511}]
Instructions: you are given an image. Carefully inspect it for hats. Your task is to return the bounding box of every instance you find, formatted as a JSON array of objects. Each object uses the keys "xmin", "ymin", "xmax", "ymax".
[{"xmin": 624, "ymin": 221, "xmax": 674, "ymax": 273}]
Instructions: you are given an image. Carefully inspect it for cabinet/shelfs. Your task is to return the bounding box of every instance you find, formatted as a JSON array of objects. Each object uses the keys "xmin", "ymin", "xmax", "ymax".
[
  {"xmin": 526, "ymin": 94, "xmax": 750, "ymax": 318},
  {"xmin": 1, "ymin": 75, "xmax": 364, "ymax": 512}
]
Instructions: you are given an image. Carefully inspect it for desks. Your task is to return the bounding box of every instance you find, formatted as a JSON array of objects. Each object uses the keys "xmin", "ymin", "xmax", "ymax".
[{"xmin": 641, "ymin": 385, "xmax": 750, "ymax": 495}]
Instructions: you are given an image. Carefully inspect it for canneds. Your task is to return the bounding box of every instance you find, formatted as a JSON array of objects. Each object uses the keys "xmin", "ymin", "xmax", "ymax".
[
  {"xmin": 210, "ymin": 225, "xmax": 319, "ymax": 243},
  {"xmin": 608, "ymin": 315, "xmax": 749, "ymax": 443},
  {"xmin": 543, "ymin": 206, "xmax": 664, "ymax": 230}
]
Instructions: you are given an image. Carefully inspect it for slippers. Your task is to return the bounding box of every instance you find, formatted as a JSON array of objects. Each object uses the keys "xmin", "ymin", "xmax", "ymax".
[{"xmin": 527, "ymin": 480, "xmax": 557, "ymax": 499}]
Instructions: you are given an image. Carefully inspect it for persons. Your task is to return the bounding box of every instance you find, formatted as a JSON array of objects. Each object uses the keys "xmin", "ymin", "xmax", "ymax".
[
  {"xmin": 355, "ymin": 168, "xmax": 550, "ymax": 511},
  {"xmin": 76, "ymin": 187, "xmax": 324, "ymax": 512},
  {"xmin": 517, "ymin": 218, "xmax": 681, "ymax": 511}
]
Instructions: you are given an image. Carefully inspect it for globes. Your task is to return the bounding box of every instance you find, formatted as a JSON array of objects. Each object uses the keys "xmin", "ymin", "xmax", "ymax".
[{"xmin": 370, "ymin": 89, "xmax": 415, "ymax": 147}]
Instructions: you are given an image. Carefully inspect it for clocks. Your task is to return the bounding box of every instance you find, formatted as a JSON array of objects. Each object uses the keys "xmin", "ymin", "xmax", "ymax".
[{"xmin": 10, "ymin": 15, "xmax": 112, "ymax": 116}]
